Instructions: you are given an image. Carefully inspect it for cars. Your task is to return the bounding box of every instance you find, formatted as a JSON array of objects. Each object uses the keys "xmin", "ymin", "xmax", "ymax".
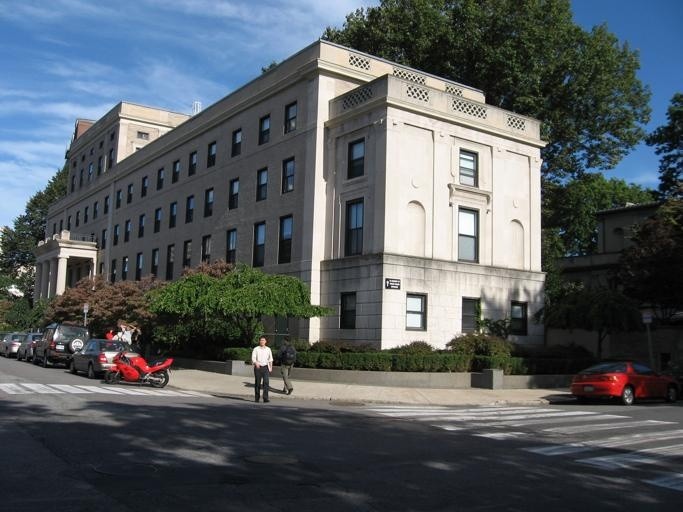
[
  {"xmin": 69, "ymin": 339, "xmax": 140, "ymax": 379},
  {"xmin": 570, "ymin": 360, "xmax": 683, "ymax": 405},
  {"xmin": 1, "ymin": 332, "xmax": 43, "ymax": 363}
]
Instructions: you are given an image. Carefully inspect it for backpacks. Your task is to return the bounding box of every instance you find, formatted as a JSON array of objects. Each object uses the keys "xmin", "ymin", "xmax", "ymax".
[{"xmin": 282, "ymin": 345, "xmax": 295, "ymax": 364}]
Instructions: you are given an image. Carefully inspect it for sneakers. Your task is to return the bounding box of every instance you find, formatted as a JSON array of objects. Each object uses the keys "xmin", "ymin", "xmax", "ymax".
[
  {"xmin": 264, "ymin": 398, "xmax": 269, "ymax": 402},
  {"xmin": 255, "ymin": 399, "xmax": 259, "ymax": 402},
  {"xmin": 283, "ymin": 388, "xmax": 293, "ymax": 395}
]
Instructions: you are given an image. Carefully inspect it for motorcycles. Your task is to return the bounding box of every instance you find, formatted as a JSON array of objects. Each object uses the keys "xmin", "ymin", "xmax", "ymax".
[{"xmin": 104, "ymin": 348, "xmax": 174, "ymax": 388}]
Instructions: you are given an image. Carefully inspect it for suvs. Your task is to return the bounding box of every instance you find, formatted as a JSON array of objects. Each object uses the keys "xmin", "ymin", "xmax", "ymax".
[{"xmin": 33, "ymin": 323, "xmax": 91, "ymax": 371}]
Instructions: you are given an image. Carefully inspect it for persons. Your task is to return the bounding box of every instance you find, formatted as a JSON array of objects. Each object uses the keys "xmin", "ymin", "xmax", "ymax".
[
  {"xmin": 251, "ymin": 335, "xmax": 273, "ymax": 402},
  {"xmin": 105, "ymin": 325, "xmax": 143, "ymax": 346},
  {"xmin": 277, "ymin": 336, "xmax": 296, "ymax": 395}
]
